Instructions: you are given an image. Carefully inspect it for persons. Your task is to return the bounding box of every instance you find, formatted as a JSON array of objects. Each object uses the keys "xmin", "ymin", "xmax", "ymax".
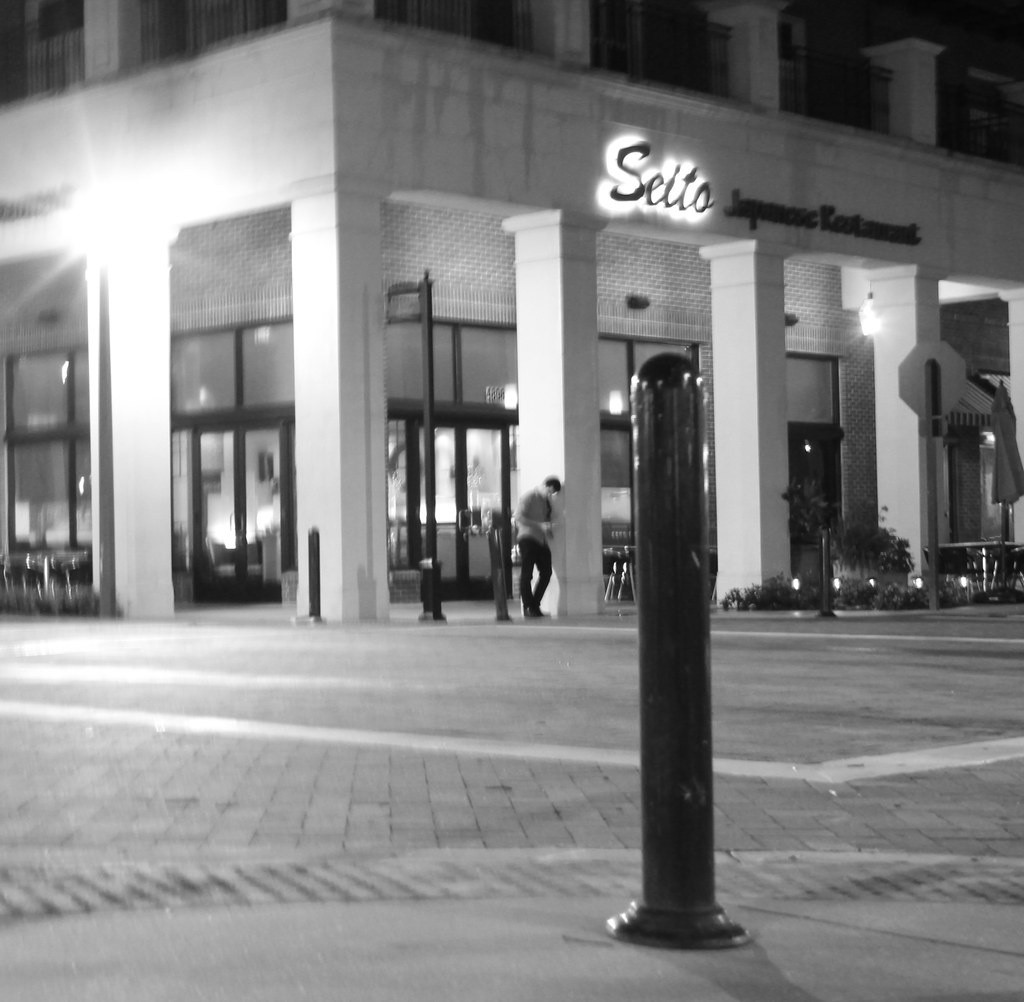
[{"xmin": 515, "ymin": 477, "xmax": 562, "ymax": 616}]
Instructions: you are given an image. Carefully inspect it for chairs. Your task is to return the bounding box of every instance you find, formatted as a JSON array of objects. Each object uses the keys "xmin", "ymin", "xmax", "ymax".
[{"xmin": 924, "ymin": 539, "xmax": 1024, "ymax": 604}]
